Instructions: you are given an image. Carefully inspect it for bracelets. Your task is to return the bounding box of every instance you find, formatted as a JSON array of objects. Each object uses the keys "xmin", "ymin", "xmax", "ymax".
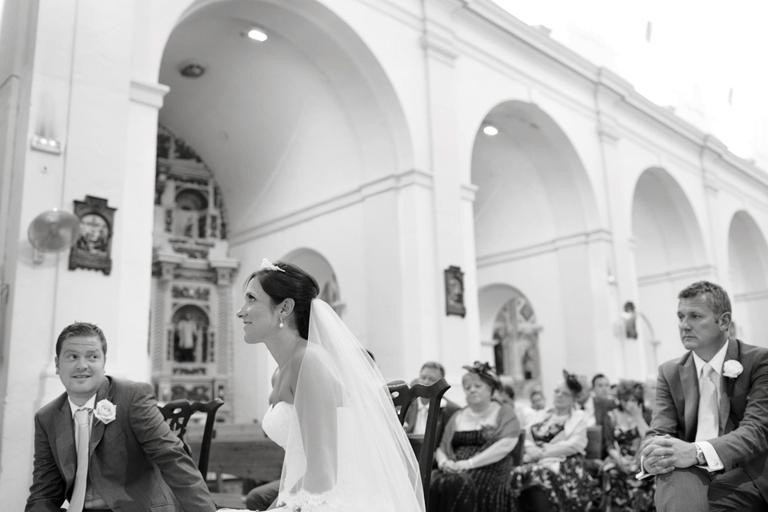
[{"xmin": 469, "ymin": 456, "xmax": 475, "ymax": 470}]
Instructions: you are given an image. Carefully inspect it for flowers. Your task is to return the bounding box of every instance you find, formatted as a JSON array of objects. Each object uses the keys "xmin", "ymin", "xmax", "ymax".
[
  {"xmin": 94, "ymin": 399, "xmax": 118, "ymax": 423},
  {"xmin": 722, "ymin": 360, "xmax": 744, "ymax": 380}
]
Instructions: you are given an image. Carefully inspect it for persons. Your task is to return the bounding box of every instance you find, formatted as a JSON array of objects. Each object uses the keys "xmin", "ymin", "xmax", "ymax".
[
  {"xmin": 23, "ymin": 321, "xmax": 219, "ymax": 511},
  {"xmin": 635, "ymin": 281, "xmax": 767, "ymax": 512},
  {"xmin": 412, "ymin": 357, "xmax": 458, "ymax": 468},
  {"xmin": 175, "ymin": 311, "xmax": 198, "ymax": 360},
  {"xmin": 496, "ymin": 369, "xmax": 659, "ymax": 511},
  {"xmin": 425, "ymin": 361, "xmax": 520, "ymax": 512},
  {"xmin": 236, "ymin": 258, "xmax": 427, "ymax": 512}
]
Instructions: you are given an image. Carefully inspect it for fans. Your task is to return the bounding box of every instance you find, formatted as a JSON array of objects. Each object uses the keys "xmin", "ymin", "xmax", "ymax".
[{"xmin": 28, "ymin": 207, "xmax": 81, "ymax": 267}]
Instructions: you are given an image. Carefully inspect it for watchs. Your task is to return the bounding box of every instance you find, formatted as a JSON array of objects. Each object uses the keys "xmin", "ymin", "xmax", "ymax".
[{"xmin": 696, "ymin": 442, "xmax": 706, "ymax": 466}]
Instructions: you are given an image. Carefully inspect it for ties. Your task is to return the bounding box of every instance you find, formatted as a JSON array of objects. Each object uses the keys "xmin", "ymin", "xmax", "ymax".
[
  {"xmin": 695, "ymin": 363, "xmax": 720, "ymax": 443},
  {"xmin": 67, "ymin": 408, "xmax": 90, "ymax": 512}
]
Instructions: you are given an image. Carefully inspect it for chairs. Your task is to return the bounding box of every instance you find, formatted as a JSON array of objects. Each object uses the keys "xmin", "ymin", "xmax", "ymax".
[
  {"xmin": 383, "ymin": 382, "xmax": 454, "ymax": 509},
  {"xmin": 156, "ymin": 399, "xmax": 221, "ymax": 482}
]
